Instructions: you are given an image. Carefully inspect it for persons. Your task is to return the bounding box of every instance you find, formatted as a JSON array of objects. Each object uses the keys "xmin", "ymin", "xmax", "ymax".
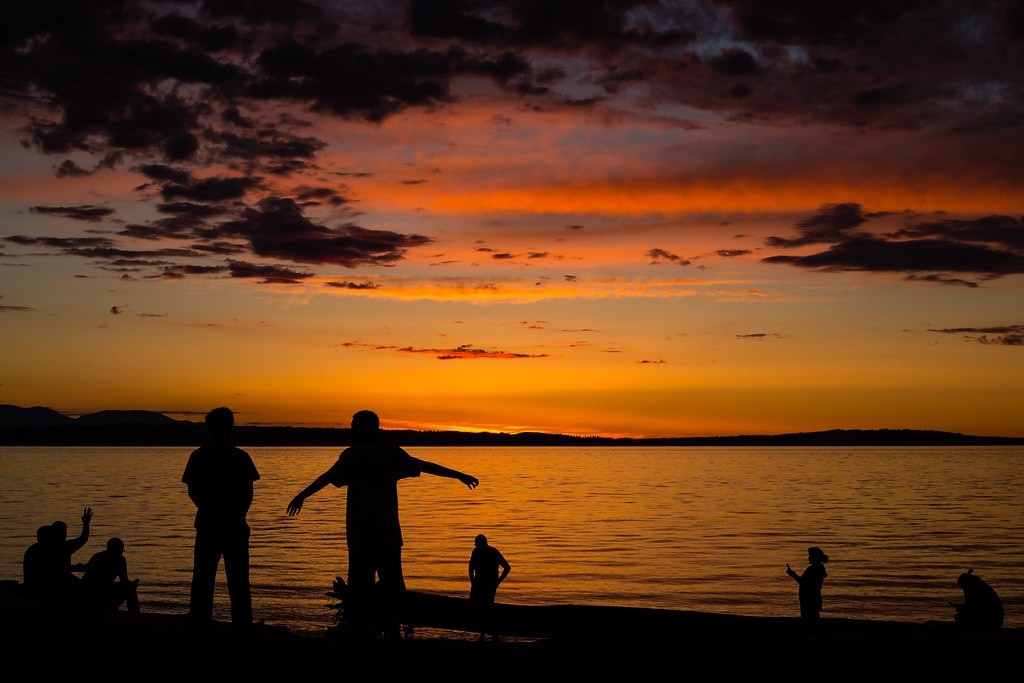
[
  {"xmin": 467, "ymin": 533, "xmax": 511, "ymax": 642},
  {"xmin": 180, "ymin": 407, "xmax": 262, "ymax": 623},
  {"xmin": 785, "ymin": 547, "xmax": 829, "ymax": 618},
  {"xmin": 955, "ymin": 568, "xmax": 1005, "ymax": 627},
  {"xmin": 80, "ymin": 537, "xmax": 141, "ymax": 614},
  {"xmin": 34, "ymin": 507, "xmax": 94, "ymax": 594},
  {"xmin": 22, "ymin": 525, "xmax": 51, "ymax": 590},
  {"xmin": 285, "ymin": 409, "xmax": 480, "ymax": 638}
]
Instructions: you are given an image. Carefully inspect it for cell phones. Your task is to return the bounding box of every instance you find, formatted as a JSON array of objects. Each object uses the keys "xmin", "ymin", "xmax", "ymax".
[{"xmin": 786, "ymin": 563, "xmax": 790, "ymax": 570}]
